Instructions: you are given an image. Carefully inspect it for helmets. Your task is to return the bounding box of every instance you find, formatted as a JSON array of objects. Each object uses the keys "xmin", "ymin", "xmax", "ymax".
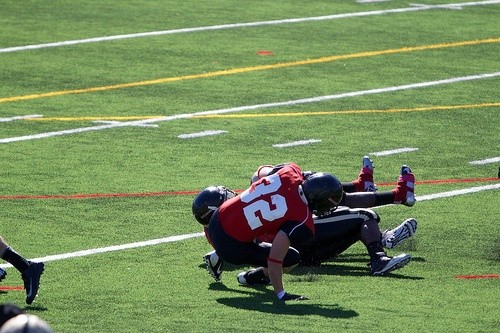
[
  {"xmin": 192, "ymin": 185, "xmax": 239, "ymax": 226},
  {"xmin": 302, "ymin": 172, "xmax": 343, "ymax": 216}
]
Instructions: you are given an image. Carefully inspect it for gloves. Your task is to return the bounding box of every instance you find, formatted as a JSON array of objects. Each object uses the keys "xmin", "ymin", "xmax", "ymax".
[{"xmin": 276, "ymin": 290, "xmax": 309, "ymax": 300}]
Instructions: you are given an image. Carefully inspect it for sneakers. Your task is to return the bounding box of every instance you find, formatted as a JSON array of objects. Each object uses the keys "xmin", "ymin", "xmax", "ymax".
[
  {"xmin": 359, "ymin": 155, "xmax": 378, "ymax": 192},
  {"xmin": 202, "ymin": 249, "xmax": 222, "ymax": 281},
  {"xmin": 397, "ymin": 165, "xmax": 416, "ymax": 207},
  {"xmin": 21, "ymin": 261, "xmax": 44, "ymax": 305},
  {"xmin": 383, "ymin": 217, "xmax": 417, "ymax": 248},
  {"xmin": 237, "ymin": 268, "xmax": 272, "ymax": 284},
  {"xmin": 371, "ymin": 253, "xmax": 411, "ymax": 276}
]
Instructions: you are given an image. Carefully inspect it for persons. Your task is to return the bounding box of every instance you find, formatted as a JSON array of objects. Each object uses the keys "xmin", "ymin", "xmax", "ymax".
[
  {"xmin": 249, "ymin": 154, "xmax": 416, "ymax": 210},
  {"xmin": 202, "ymin": 163, "xmax": 343, "ymax": 300},
  {"xmin": 0, "ymin": 236, "xmax": 44, "ymax": 305},
  {"xmin": 191, "ymin": 184, "xmax": 417, "ymax": 277}
]
